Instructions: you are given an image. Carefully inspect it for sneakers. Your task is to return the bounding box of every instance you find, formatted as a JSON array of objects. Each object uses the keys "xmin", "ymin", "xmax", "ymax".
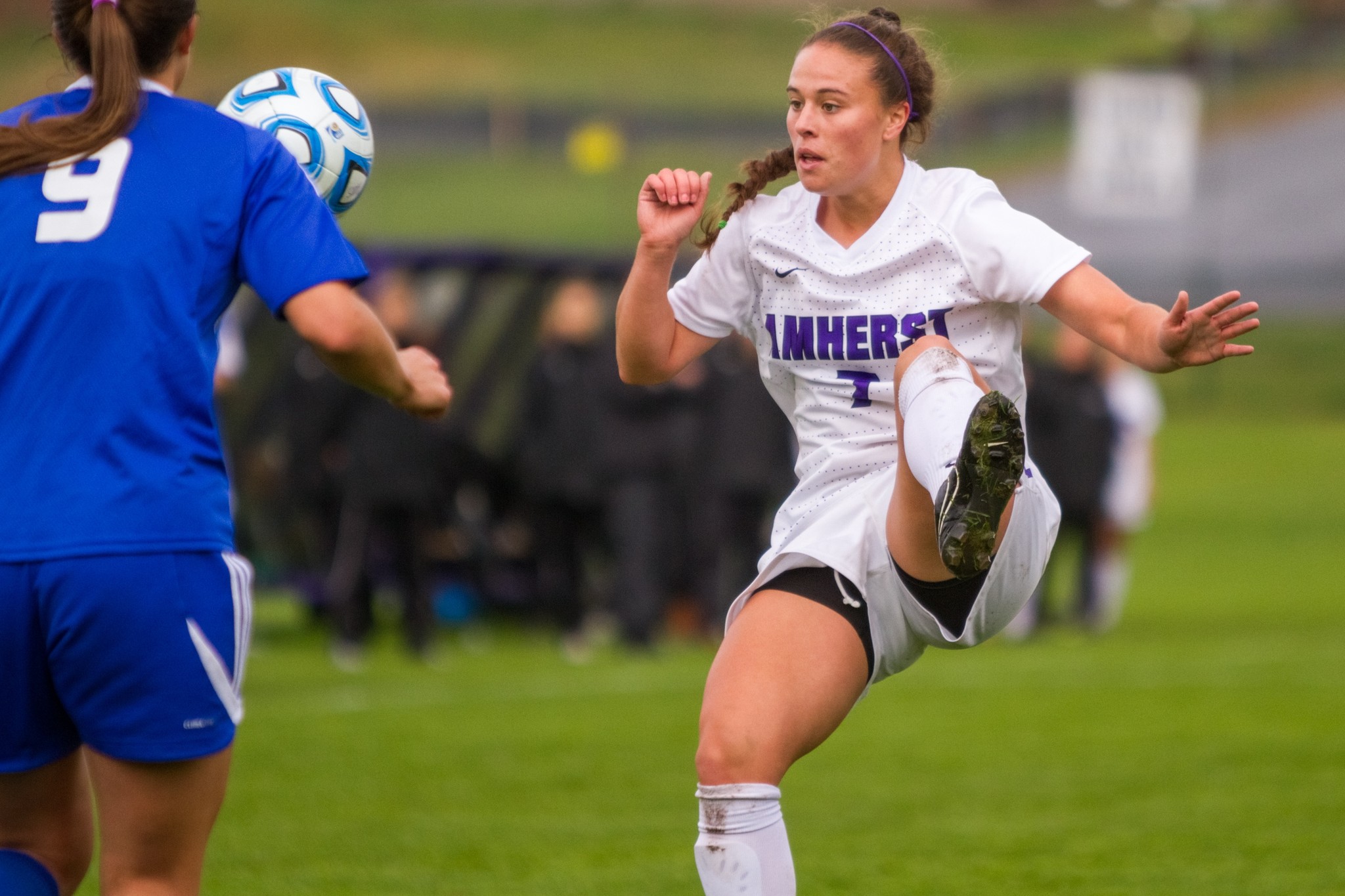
[{"xmin": 932, "ymin": 392, "xmax": 1024, "ymax": 580}]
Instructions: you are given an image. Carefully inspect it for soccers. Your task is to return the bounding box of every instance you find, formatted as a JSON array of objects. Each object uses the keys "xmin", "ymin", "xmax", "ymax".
[{"xmin": 214, "ymin": 64, "xmax": 377, "ymax": 217}]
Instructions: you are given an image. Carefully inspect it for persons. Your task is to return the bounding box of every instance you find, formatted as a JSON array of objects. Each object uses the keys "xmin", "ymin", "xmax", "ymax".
[
  {"xmin": 207, "ymin": 253, "xmax": 1162, "ymax": 674},
  {"xmin": 0, "ymin": 0, "xmax": 452, "ymax": 896},
  {"xmin": 613, "ymin": 5, "xmax": 1261, "ymax": 896}
]
